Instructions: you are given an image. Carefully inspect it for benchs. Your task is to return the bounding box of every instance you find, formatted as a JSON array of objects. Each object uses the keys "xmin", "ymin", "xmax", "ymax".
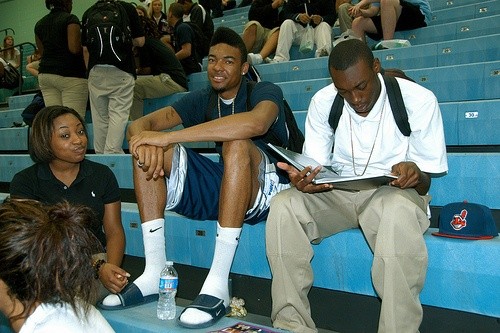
[{"xmin": 0, "ymin": 1, "xmax": 500, "ymax": 333}]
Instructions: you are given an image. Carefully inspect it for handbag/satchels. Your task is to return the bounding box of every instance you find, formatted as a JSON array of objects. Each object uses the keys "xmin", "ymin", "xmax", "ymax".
[
  {"xmin": 299, "ymin": 25, "xmax": 314, "ymax": 52},
  {"xmin": 0, "ymin": 57, "xmax": 23, "ymax": 89},
  {"xmin": 22, "ymin": 93, "xmax": 45, "ymax": 126}
]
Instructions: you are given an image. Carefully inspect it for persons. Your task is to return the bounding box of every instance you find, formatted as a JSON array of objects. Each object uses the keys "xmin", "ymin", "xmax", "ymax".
[
  {"xmin": 98, "ymin": 26, "xmax": 289, "ymax": 328},
  {"xmin": 265, "ymin": 38, "xmax": 448, "ymax": 333},
  {"xmin": 0, "ymin": 0, "xmax": 431, "ymax": 154},
  {"xmin": 0, "ymin": 194, "xmax": 115, "ymax": 333},
  {"xmin": 11, "ymin": 104, "xmax": 130, "ymax": 306}
]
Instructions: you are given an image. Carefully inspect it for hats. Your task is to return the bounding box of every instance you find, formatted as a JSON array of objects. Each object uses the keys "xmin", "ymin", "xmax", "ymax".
[
  {"xmin": 432, "ymin": 199, "xmax": 498, "ymax": 240},
  {"xmin": 211, "ymin": 26, "xmax": 248, "ymax": 59}
]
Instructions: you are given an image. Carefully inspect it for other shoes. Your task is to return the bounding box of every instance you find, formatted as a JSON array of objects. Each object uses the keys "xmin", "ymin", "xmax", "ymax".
[
  {"xmin": 247, "ymin": 53, "xmax": 263, "ymax": 65},
  {"xmin": 269, "ymin": 56, "xmax": 284, "ymax": 63}
]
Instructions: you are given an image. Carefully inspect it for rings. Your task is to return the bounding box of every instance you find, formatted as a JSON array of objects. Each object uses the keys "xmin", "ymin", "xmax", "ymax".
[{"xmin": 137, "ymin": 160, "xmax": 144, "ymax": 167}]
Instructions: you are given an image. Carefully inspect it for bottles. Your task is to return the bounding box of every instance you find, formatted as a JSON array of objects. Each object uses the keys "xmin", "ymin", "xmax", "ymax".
[
  {"xmin": 380, "ymin": 40, "xmax": 411, "ymax": 49},
  {"xmin": 157, "ymin": 260, "xmax": 179, "ymax": 320}
]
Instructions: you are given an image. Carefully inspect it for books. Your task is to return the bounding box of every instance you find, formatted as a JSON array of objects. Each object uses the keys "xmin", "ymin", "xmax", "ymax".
[{"xmin": 268, "ymin": 143, "xmax": 399, "ymax": 191}]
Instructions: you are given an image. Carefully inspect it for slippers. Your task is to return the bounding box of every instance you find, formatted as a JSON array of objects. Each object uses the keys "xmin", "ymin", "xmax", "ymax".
[
  {"xmin": 96, "ymin": 278, "xmax": 160, "ymax": 310},
  {"xmin": 178, "ymin": 294, "xmax": 231, "ymax": 329}
]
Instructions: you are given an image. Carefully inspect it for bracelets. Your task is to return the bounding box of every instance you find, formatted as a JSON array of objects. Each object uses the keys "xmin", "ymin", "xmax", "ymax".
[{"xmin": 93, "ymin": 258, "xmax": 105, "ymax": 280}]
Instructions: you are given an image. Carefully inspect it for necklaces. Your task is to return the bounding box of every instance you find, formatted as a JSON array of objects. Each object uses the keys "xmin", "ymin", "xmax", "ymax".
[
  {"xmin": 218, "ymin": 92, "xmax": 234, "ymax": 118},
  {"xmin": 350, "ymin": 96, "xmax": 386, "ymax": 177}
]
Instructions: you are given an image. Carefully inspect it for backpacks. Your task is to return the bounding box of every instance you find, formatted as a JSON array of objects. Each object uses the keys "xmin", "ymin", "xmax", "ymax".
[
  {"xmin": 80, "ymin": 0, "xmax": 132, "ymax": 64},
  {"xmin": 328, "ymin": 67, "xmax": 416, "ymax": 138}
]
